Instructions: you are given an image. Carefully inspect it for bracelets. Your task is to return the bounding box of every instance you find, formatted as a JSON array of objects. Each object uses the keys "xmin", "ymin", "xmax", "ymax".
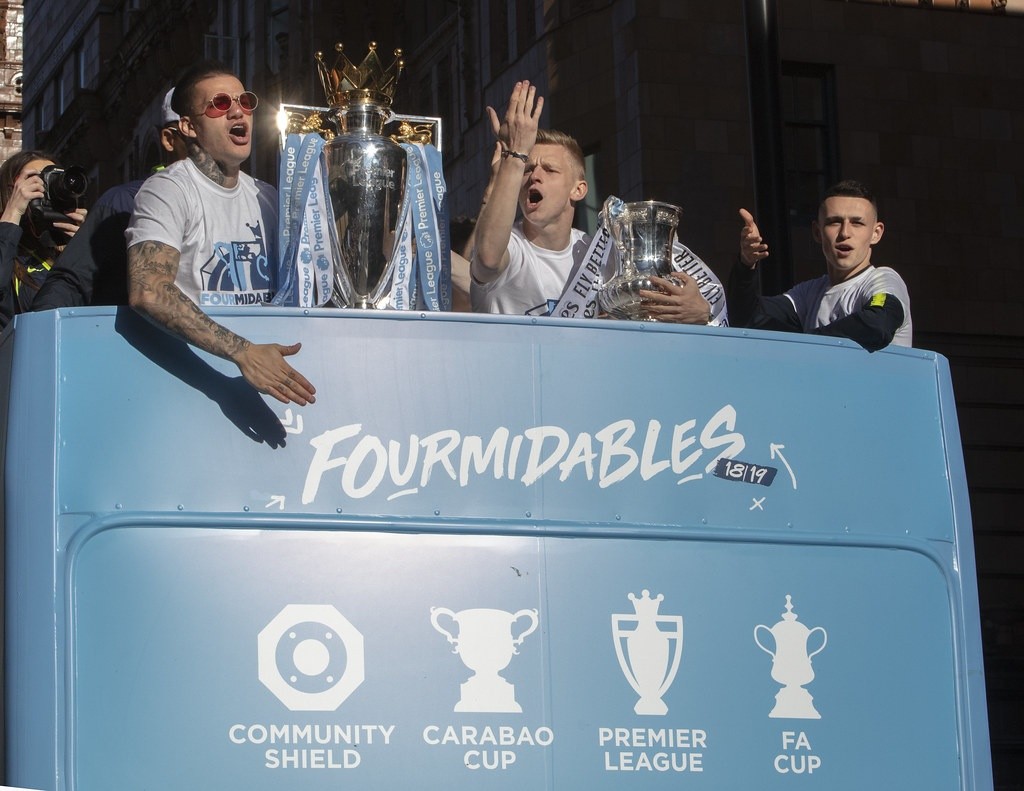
[{"xmin": 501, "ymin": 148, "xmax": 527, "ymax": 163}]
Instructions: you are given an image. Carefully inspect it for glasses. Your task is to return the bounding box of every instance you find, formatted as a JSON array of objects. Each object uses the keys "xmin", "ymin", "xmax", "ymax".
[
  {"xmin": 169, "ymin": 126, "xmax": 191, "ymax": 143},
  {"xmin": 187, "ymin": 92, "xmax": 259, "ymax": 113}
]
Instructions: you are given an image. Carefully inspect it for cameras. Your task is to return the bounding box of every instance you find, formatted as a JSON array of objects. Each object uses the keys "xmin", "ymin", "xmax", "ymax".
[{"xmin": 27, "ymin": 165, "xmax": 88, "ymax": 206}]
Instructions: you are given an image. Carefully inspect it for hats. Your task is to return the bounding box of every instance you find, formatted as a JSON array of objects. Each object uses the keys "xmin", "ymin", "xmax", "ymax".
[{"xmin": 162, "ymin": 88, "xmax": 181, "ymax": 123}]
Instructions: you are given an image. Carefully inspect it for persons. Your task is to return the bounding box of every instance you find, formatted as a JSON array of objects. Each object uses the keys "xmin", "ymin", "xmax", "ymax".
[
  {"xmin": 726, "ymin": 177, "xmax": 914, "ymax": 352},
  {"xmin": 471, "ymin": 80, "xmax": 711, "ymax": 327},
  {"xmin": 32, "ymin": 85, "xmax": 190, "ymax": 309},
  {"xmin": 0, "ymin": 150, "xmax": 88, "ymax": 332},
  {"xmin": 464, "ymin": 105, "xmax": 503, "ymax": 264},
  {"xmin": 126, "ymin": 57, "xmax": 316, "ymax": 407}
]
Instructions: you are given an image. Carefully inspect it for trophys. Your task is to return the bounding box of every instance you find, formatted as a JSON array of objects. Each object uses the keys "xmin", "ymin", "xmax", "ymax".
[
  {"xmin": 276, "ymin": 41, "xmax": 442, "ymax": 310},
  {"xmin": 596, "ymin": 196, "xmax": 683, "ymax": 323}
]
